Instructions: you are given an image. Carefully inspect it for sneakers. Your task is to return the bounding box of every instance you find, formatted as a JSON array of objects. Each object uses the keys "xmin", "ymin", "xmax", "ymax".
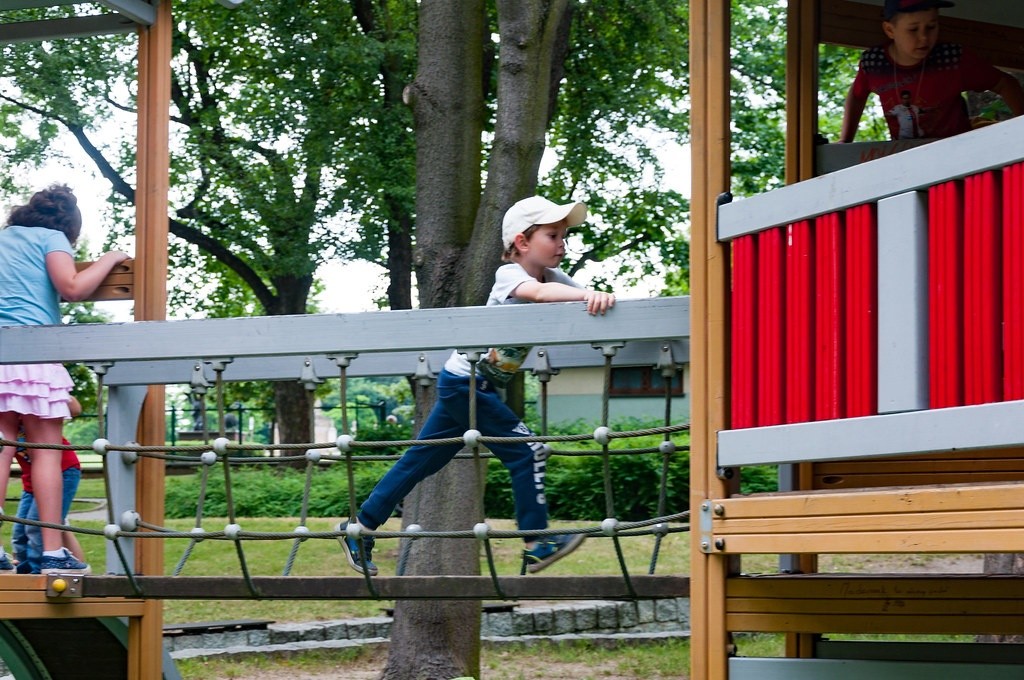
[
  {"xmin": 334, "ymin": 518, "xmax": 378, "ymax": 575},
  {"xmin": 41, "ymin": 547, "xmax": 90, "ymax": 574},
  {"xmin": 523, "ymin": 533, "xmax": 584, "ymax": 573},
  {"xmin": 0, "ymin": 553, "xmax": 17, "ymax": 574}
]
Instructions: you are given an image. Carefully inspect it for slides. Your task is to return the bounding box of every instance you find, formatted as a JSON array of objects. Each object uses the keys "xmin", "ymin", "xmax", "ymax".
[{"xmin": 0, "ymin": 617, "xmax": 184, "ymax": 679}]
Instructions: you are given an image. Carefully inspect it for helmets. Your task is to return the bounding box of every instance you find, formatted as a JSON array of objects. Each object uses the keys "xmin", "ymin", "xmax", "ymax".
[{"xmin": 502, "ymin": 196, "xmax": 587, "ymax": 252}]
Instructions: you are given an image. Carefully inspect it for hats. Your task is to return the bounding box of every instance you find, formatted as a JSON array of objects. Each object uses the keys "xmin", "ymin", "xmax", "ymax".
[{"xmin": 884, "ymin": 0, "xmax": 955, "ymax": 20}]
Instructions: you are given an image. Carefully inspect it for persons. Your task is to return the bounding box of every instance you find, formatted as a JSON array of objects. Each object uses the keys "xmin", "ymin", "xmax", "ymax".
[
  {"xmin": 14, "ymin": 421, "xmax": 81, "ymax": 574},
  {"xmin": 333, "ymin": 195, "xmax": 615, "ymax": 577},
  {"xmin": 0, "ymin": 182, "xmax": 131, "ymax": 577},
  {"xmin": 836, "ymin": 0, "xmax": 1024, "ymax": 144}
]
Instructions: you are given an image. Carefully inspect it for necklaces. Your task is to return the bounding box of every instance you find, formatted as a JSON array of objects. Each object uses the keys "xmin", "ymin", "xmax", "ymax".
[{"xmin": 893, "ymin": 46, "xmax": 924, "ymax": 103}]
